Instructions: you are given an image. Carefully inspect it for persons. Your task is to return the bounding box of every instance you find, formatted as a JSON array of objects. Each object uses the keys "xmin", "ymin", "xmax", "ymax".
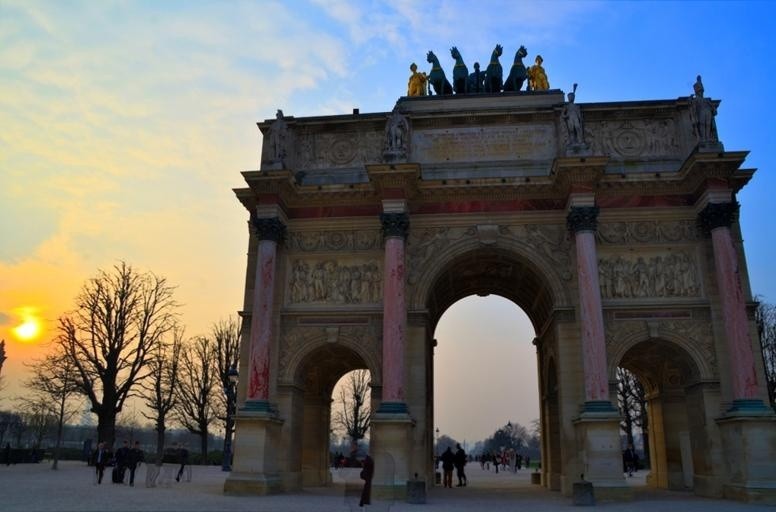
[
  {"xmin": 408, "ymin": 62, "xmax": 429, "ymax": 95},
  {"xmin": 359, "ymin": 455, "xmax": 375, "ymax": 508},
  {"xmin": 624, "ymin": 443, "xmax": 636, "ymax": 478},
  {"xmin": 335, "ymin": 450, "xmax": 339, "ymax": 470},
  {"xmin": 597, "ymin": 253, "xmax": 699, "ymax": 297},
  {"xmin": 339, "ymin": 451, "xmax": 345, "ymax": 471},
  {"xmin": 469, "ymin": 61, "xmax": 484, "ymax": 93},
  {"xmin": 266, "ymin": 108, "xmax": 293, "ymax": 163},
  {"xmin": 382, "ymin": 104, "xmax": 409, "ymax": 159},
  {"xmin": 528, "ymin": 54, "xmax": 549, "ymax": 90},
  {"xmin": 465, "ymin": 448, "xmax": 530, "ymax": 475},
  {"xmin": 688, "ymin": 76, "xmax": 716, "ymax": 147},
  {"xmin": 562, "ymin": 92, "xmax": 585, "ymax": 145},
  {"xmin": 1, "ymin": 438, "xmax": 192, "ymax": 489},
  {"xmin": 536, "ymin": 468, "xmax": 538, "ymax": 472},
  {"xmin": 285, "ymin": 261, "xmax": 382, "ymax": 305},
  {"xmin": 538, "ymin": 462, "xmax": 542, "ymax": 473},
  {"xmin": 440, "ymin": 446, "xmax": 454, "ymax": 489},
  {"xmin": 454, "ymin": 443, "xmax": 466, "ymax": 487}
]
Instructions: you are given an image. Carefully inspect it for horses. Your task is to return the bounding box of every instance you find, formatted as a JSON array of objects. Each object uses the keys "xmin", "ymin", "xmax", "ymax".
[
  {"xmin": 447, "ymin": 47, "xmax": 470, "ymax": 95},
  {"xmin": 486, "ymin": 44, "xmax": 504, "ymax": 92},
  {"xmin": 423, "ymin": 50, "xmax": 454, "ymax": 97},
  {"xmin": 504, "ymin": 45, "xmax": 533, "ymax": 89}
]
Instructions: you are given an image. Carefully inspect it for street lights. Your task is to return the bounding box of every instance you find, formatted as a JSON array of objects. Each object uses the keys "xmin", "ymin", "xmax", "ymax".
[
  {"xmin": 50, "ymin": 357, "xmax": 74, "ymax": 470},
  {"xmin": 435, "ymin": 427, "xmax": 440, "ymax": 458}
]
